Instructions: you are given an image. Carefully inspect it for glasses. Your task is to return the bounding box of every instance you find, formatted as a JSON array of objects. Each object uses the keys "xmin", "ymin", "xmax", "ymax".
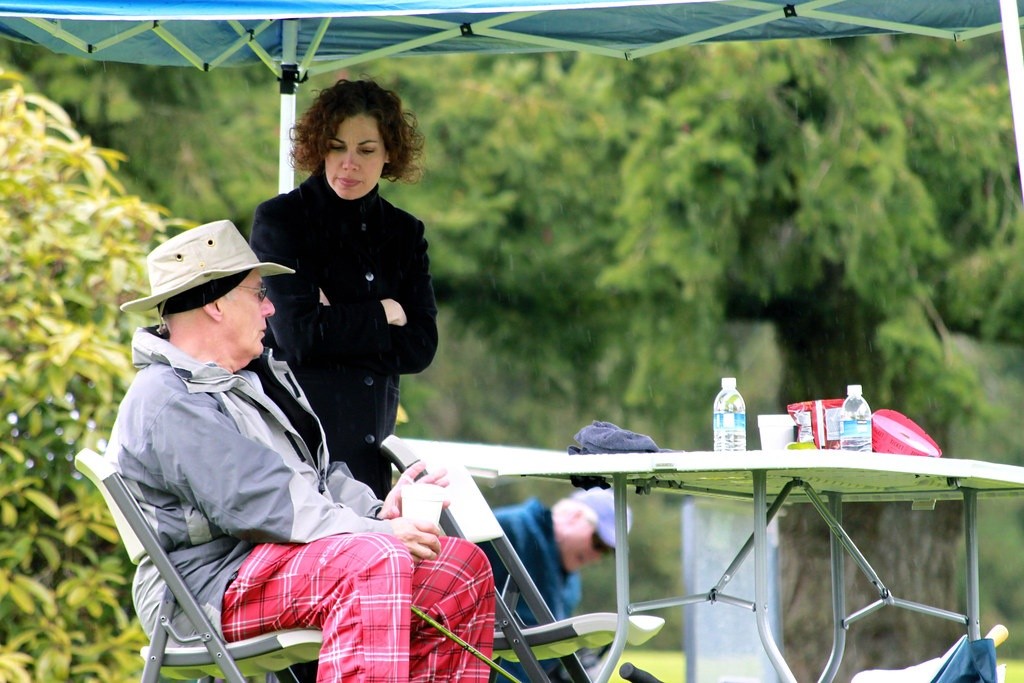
[
  {"xmin": 234, "ymin": 284, "xmax": 268, "ymax": 301},
  {"xmin": 588, "ymin": 519, "xmax": 610, "ymax": 553}
]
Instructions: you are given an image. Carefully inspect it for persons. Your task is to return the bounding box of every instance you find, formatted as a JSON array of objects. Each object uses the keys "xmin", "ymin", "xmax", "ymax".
[
  {"xmin": 105, "ymin": 232, "xmax": 494, "ymax": 683},
  {"xmin": 253, "ymin": 77, "xmax": 440, "ymax": 508},
  {"xmin": 483, "ymin": 483, "xmax": 631, "ymax": 683}
]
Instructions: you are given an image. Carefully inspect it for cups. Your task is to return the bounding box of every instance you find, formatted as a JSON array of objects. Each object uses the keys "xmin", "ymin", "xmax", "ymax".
[
  {"xmin": 400, "ymin": 481, "xmax": 446, "ymax": 527},
  {"xmin": 757, "ymin": 414, "xmax": 800, "ymax": 450}
]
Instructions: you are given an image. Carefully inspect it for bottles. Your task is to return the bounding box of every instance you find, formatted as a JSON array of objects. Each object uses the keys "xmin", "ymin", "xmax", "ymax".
[
  {"xmin": 840, "ymin": 384, "xmax": 873, "ymax": 452},
  {"xmin": 713, "ymin": 377, "xmax": 747, "ymax": 451}
]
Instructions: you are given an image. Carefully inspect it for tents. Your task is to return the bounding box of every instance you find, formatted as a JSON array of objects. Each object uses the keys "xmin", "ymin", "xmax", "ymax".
[{"xmin": 0, "ymin": 0, "xmax": 1023, "ymax": 683}]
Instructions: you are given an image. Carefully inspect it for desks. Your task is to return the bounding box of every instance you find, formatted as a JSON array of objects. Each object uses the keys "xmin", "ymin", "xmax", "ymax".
[{"xmin": 494, "ymin": 445, "xmax": 1023, "ymax": 683}]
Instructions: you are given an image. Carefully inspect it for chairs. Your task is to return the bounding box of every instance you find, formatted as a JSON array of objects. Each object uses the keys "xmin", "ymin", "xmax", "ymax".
[
  {"xmin": 379, "ymin": 431, "xmax": 666, "ymax": 683},
  {"xmin": 73, "ymin": 445, "xmax": 324, "ymax": 683}
]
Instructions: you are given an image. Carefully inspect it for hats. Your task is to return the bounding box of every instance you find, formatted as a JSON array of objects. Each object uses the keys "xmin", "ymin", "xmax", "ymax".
[
  {"xmin": 119, "ymin": 218, "xmax": 297, "ymax": 312},
  {"xmin": 570, "ymin": 483, "xmax": 633, "ymax": 549}
]
